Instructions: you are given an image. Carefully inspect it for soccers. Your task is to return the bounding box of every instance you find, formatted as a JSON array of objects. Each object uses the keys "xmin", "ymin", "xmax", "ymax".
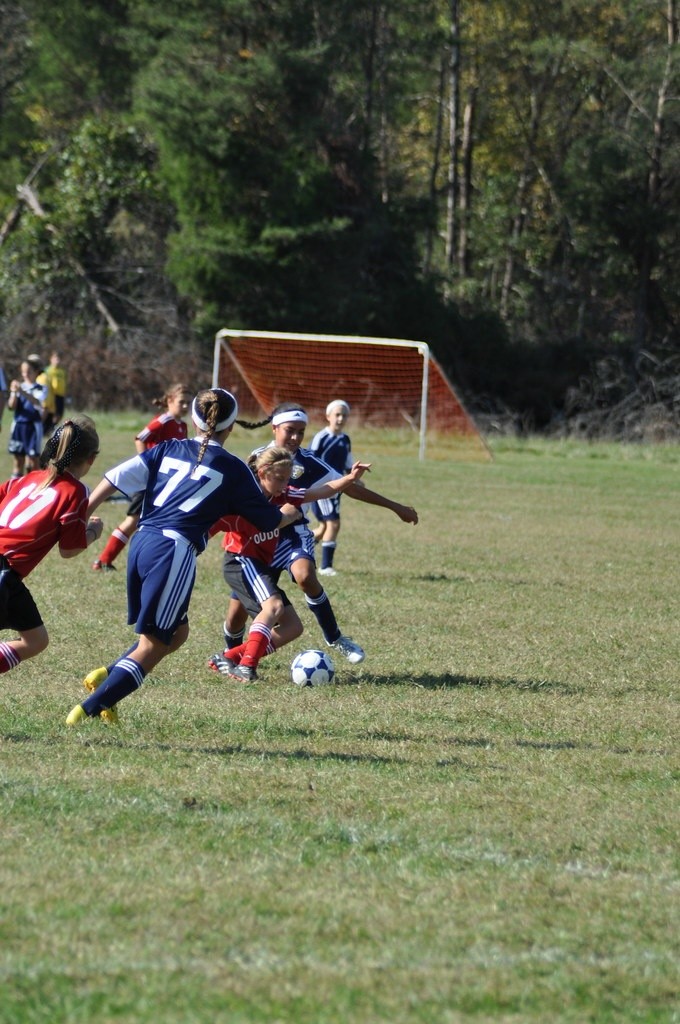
[{"xmin": 291, "ymin": 650, "xmax": 337, "ymax": 688}]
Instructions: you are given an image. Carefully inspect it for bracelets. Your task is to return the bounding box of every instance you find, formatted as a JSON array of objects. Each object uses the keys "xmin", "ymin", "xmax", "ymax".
[{"xmin": 85, "ymin": 528, "xmax": 97, "ymax": 541}]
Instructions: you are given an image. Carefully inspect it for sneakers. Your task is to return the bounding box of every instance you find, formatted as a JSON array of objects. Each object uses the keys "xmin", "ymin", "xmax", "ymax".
[
  {"xmin": 324, "ymin": 635, "xmax": 364, "ymax": 664},
  {"xmin": 229, "ymin": 665, "xmax": 259, "ymax": 684},
  {"xmin": 66, "ymin": 705, "xmax": 87, "ymax": 725},
  {"xmin": 207, "ymin": 652, "xmax": 236, "ymax": 676},
  {"xmin": 84, "ymin": 667, "xmax": 118, "ymax": 728}
]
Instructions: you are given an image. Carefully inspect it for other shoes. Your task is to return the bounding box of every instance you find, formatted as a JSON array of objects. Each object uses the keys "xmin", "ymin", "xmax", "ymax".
[
  {"xmin": 92, "ymin": 560, "xmax": 118, "ymax": 571},
  {"xmin": 318, "ymin": 566, "xmax": 337, "ymax": 576}
]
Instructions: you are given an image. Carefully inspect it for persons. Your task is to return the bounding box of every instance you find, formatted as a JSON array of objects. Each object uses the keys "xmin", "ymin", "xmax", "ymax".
[
  {"xmin": 66, "ymin": 376, "xmax": 418, "ymax": 730},
  {"xmin": 0, "ymin": 351, "xmax": 67, "ymax": 480},
  {"xmin": 0, "ymin": 413, "xmax": 103, "ymax": 673}
]
